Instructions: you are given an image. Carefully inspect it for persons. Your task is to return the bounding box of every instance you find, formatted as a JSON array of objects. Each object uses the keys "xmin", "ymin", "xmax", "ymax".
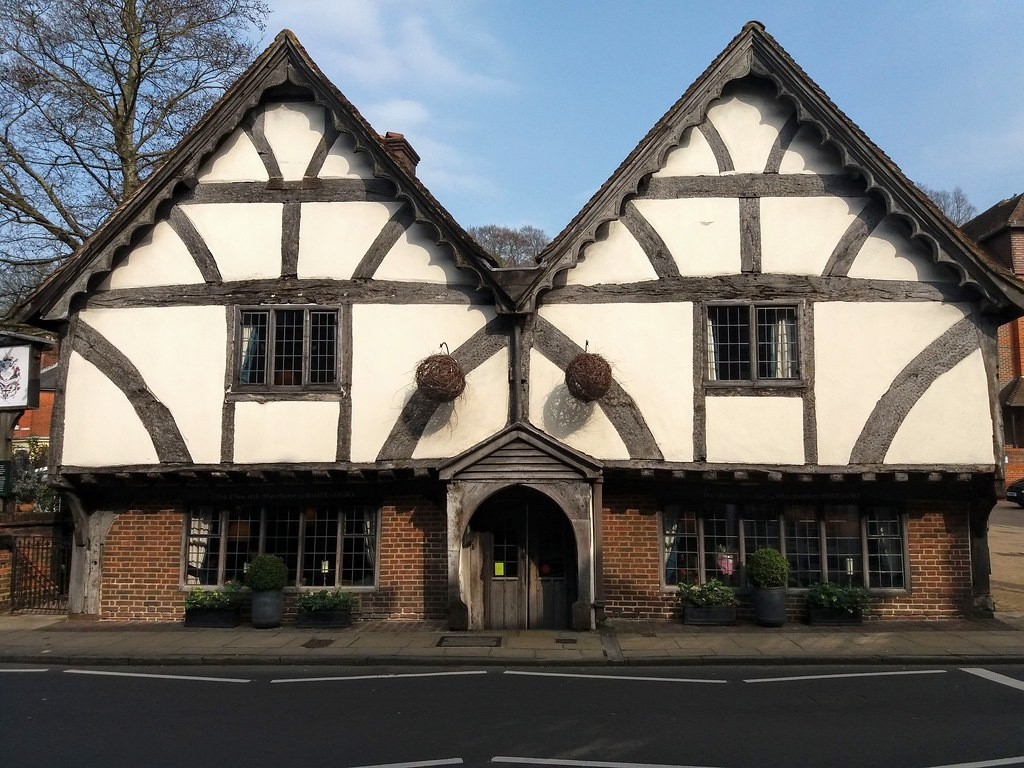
[{"xmin": 717, "ymin": 534, "xmax": 739, "ymax": 586}]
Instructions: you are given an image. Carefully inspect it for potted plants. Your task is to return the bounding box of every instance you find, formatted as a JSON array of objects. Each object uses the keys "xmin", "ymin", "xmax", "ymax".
[
  {"xmin": 244, "ymin": 554, "xmax": 289, "ymax": 628},
  {"xmin": 11, "ymin": 431, "xmax": 52, "ymax": 512},
  {"xmin": 747, "ymin": 549, "xmax": 795, "ymax": 628},
  {"xmin": 291, "ymin": 578, "xmax": 353, "ymax": 628},
  {"xmin": 678, "ymin": 578, "xmax": 743, "ymax": 625},
  {"xmin": 185, "ymin": 580, "xmax": 248, "ymax": 629},
  {"xmin": 804, "ymin": 582, "xmax": 870, "ymax": 626}
]
